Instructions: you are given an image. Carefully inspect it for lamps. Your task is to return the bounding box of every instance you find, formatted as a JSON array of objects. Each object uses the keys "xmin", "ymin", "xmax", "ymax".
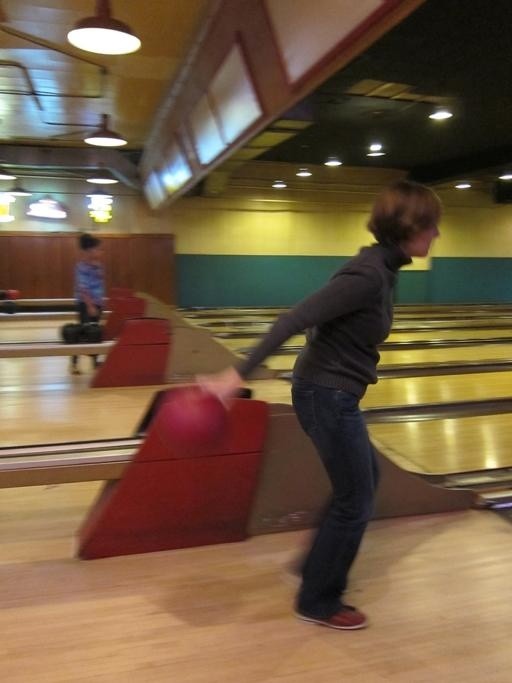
[
  {"xmin": 68, "ymin": 1, "xmax": 142, "ymax": 56},
  {"xmin": 83, "ymin": 113, "xmax": 128, "ymax": 147}
]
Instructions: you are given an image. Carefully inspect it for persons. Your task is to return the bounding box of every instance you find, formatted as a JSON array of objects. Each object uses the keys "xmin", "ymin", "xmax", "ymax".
[
  {"xmin": 197, "ymin": 178, "xmax": 443, "ymax": 630},
  {"xmin": 69, "ymin": 233, "xmax": 105, "ymax": 375}
]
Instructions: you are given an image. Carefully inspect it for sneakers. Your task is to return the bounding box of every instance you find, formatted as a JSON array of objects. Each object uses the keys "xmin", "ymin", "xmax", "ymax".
[
  {"xmin": 291, "ymin": 600, "xmax": 369, "ymax": 631},
  {"xmin": 66, "ymin": 362, "xmax": 81, "ymax": 375}
]
